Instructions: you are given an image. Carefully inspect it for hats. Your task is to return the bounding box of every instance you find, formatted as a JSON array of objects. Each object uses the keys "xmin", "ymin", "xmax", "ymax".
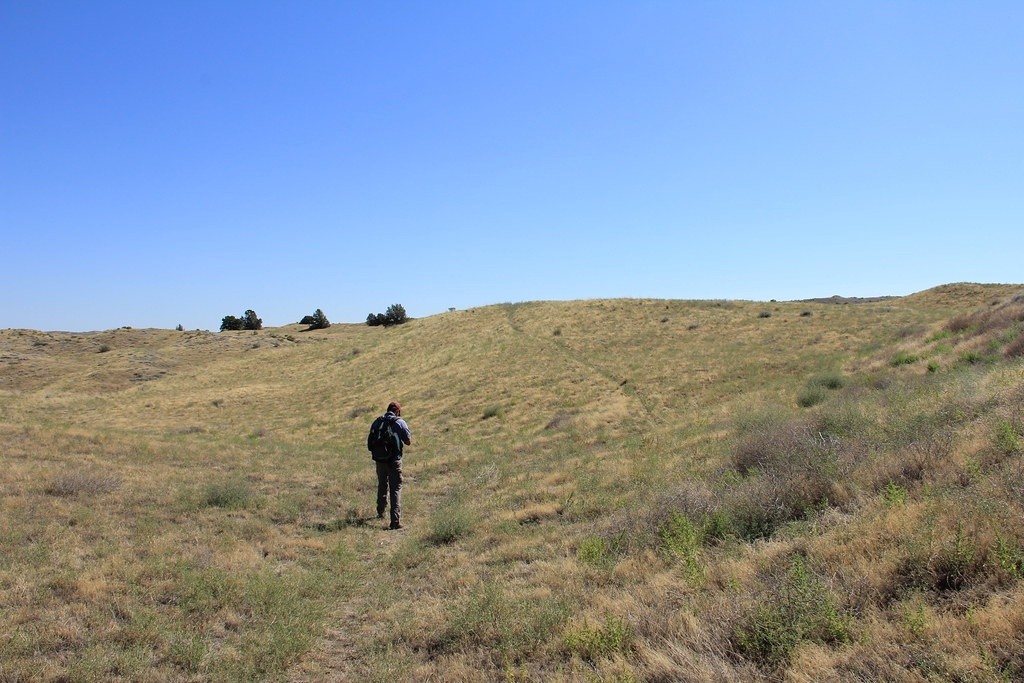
[{"xmin": 388, "ymin": 402, "xmax": 401, "ymax": 416}]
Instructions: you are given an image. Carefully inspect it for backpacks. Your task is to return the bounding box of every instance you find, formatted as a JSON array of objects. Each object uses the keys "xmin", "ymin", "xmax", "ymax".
[{"xmin": 368, "ymin": 416, "xmax": 401, "ymax": 458}]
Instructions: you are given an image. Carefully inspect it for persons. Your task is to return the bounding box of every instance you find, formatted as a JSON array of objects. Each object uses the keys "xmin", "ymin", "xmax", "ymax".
[{"xmin": 367, "ymin": 402, "xmax": 411, "ymax": 529}]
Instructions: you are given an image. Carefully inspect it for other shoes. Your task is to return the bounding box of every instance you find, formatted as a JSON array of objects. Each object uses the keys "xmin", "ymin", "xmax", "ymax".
[
  {"xmin": 390, "ymin": 522, "xmax": 404, "ymax": 529},
  {"xmin": 377, "ymin": 511, "xmax": 386, "ymax": 518}
]
